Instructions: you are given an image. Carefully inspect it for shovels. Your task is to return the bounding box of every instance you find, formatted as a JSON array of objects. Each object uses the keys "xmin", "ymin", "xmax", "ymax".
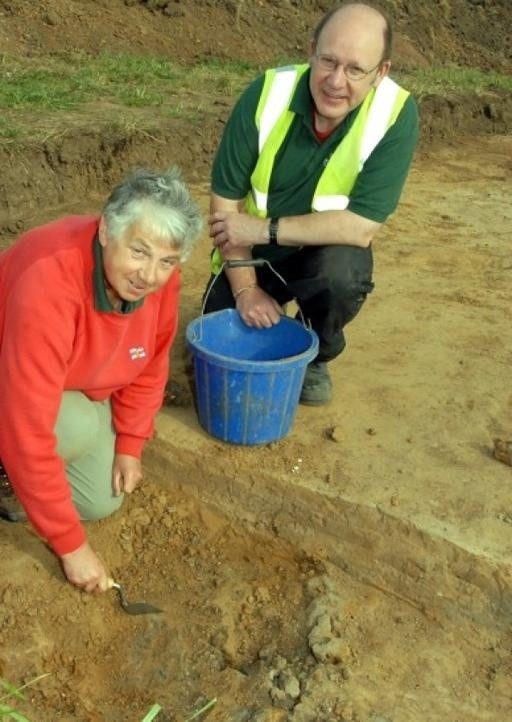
[{"xmin": 107, "ymin": 577, "xmax": 164, "ymax": 615}]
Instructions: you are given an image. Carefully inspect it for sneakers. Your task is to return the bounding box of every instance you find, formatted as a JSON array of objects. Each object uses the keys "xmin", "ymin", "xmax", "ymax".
[
  {"xmin": 299, "ymin": 359, "xmax": 336, "ymax": 407},
  {"xmin": 0, "ymin": 462, "xmax": 31, "ymax": 523}
]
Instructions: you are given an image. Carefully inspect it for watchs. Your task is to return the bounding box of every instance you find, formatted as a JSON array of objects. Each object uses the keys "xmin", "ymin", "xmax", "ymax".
[{"xmin": 270, "ymin": 216, "xmax": 278, "ymax": 247}]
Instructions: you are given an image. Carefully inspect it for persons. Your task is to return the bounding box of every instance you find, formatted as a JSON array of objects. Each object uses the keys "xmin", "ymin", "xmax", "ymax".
[
  {"xmin": 1, "ymin": 166, "xmax": 203, "ymax": 597},
  {"xmin": 200, "ymin": 3, "xmax": 421, "ymax": 411}
]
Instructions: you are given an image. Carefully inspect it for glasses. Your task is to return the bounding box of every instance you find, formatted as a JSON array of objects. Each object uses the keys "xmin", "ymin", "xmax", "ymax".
[{"xmin": 315, "ymin": 42, "xmax": 382, "ymax": 82}]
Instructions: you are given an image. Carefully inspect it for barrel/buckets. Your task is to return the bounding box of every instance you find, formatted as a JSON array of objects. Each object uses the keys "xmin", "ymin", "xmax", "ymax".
[{"xmin": 182, "ymin": 257, "xmax": 322, "ymax": 447}]
